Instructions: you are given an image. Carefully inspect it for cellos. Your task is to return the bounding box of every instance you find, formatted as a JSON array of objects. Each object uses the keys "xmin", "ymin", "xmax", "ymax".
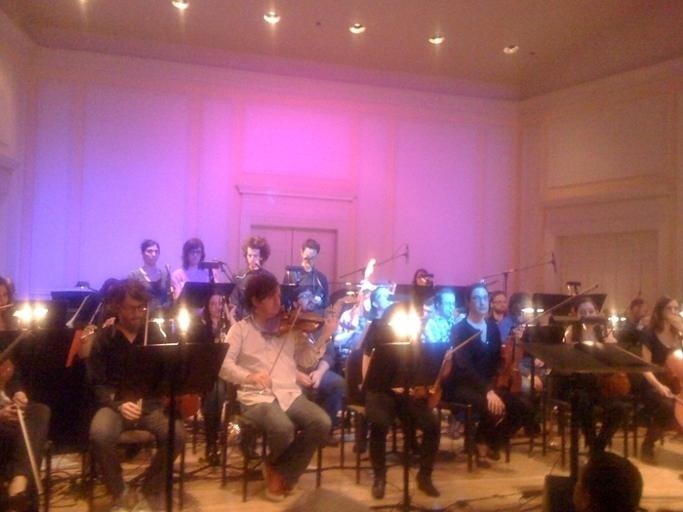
[{"xmin": 666, "ymin": 327, "xmax": 683, "ymax": 426}]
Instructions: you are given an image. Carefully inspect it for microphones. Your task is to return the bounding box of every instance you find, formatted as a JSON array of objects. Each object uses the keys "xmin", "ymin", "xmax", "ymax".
[
  {"xmin": 165, "ymin": 264, "xmax": 171, "ymax": 274},
  {"xmin": 403, "ymin": 244, "xmax": 409, "ymax": 264},
  {"xmin": 416, "ymin": 273, "xmax": 434, "ymax": 278},
  {"xmin": 551, "ymin": 252, "xmax": 558, "ymax": 272},
  {"xmin": 304, "ymin": 255, "xmax": 308, "ymax": 261}
]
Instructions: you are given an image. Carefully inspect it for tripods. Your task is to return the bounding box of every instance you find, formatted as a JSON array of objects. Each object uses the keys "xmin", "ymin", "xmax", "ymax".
[{"xmin": 371, "ymin": 389, "xmax": 429, "ymax": 511}]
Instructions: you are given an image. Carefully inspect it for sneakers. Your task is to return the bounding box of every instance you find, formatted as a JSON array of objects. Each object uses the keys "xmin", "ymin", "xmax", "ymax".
[{"xmin": 262, "ymin": 466, "xmax": 294, "ymax": 501}]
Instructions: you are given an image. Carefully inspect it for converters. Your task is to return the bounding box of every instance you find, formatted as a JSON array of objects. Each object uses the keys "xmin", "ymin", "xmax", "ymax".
[{"xmin": 523, "ymin": 490, "xmax": 541, "ymax": 498}]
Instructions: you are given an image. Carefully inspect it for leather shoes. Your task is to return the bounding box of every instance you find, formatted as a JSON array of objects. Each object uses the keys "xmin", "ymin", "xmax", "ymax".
[
  {"xmin": 419, "ymin": 479, "xmax": 439, "ymax": 496},
  {"xmin": 372, "ymin": 476, "xmax": 386, "ymax": 498}
]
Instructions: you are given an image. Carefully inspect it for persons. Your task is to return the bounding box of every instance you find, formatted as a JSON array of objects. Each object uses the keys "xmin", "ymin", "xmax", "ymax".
[
  {"xmin": 127, "ymin": 237, "xmax": 171, "ymax": 316},
  {"xmin": 189, "ymin": 267, "xmax": 347, "ymax": 502},
  {"xmin": 84, "ymin": 278, "xmax": 188, "ymax": 512},
  {"xmin": 335, "ymin": 268, "xmax": 682, "ymax": 500},
  {"xmin": 232, "ymin": 236, "xmax": 270, "ymax": 281},
  {"xmin": 0, "ymin": 355, "xmax": 53, "ymax": 511},
  {"xmin": 282, "ymin": 238, "xmax": 329, "ymax": 301},
  {"xmin": 574, "ymin": 451, "xmax": 642, "ymax": 512},
  {"xmin": 0, "ymin": 276, "xmax": 12, "ymax": 332},
  {"xmin": 171, "ymin": 237, "xmax": 217, "ymax": 317}
]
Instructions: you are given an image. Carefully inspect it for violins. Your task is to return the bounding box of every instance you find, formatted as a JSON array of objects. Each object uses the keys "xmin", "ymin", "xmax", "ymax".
[
  {"xmin": 268, "ymin": 310, "xmax": 356, "ymax": 333},
  {"xmin": 595, "ymin": 326, "xmax": 630, "ymax": 399},
  {"xmin": 495, "ymin": 316, "xmax": 522, "ymax": 395}
]
